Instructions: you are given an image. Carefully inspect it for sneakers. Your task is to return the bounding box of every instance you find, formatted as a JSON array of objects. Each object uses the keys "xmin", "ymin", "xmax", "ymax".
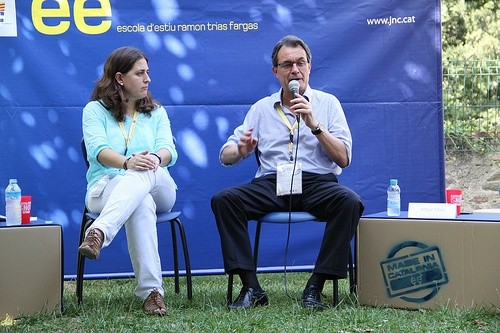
[
  {"xmin": 78, "ymin": 229, "xmax": 102, "ymax": 260},
  {"xmin": 143, "ymin": 291, "xmax": 166, "ymax": 314}
]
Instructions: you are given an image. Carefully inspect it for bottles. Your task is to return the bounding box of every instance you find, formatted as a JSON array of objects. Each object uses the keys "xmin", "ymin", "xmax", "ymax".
[
  {"xmin": 5, "ymin": 179, "xmax": 22, "ymax": 226},
  {"xmin": 387, "ymin": 179, "xmax": 401, "ymax": 216}
]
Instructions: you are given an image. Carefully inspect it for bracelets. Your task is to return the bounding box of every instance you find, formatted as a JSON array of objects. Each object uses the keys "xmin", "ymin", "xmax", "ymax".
[{"xmin": 123, "ymin": 157, "xmax": 131, "ymax": 170}]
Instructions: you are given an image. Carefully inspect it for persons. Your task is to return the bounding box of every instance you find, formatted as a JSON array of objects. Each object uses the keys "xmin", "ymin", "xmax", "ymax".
[
  {"xmin": 76, "ymin": 46, "xmax": 178, "ymax": 312},
  {"xmin": 211, "ymin": 36, "xmax": 366, "ymax": 313}
]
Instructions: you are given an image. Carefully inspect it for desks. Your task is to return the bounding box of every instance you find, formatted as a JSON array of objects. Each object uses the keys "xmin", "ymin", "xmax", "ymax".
[
  {"xmin": 0, "ymin": 223, "xmax": 65, "ymax": 324},
  {"xmin": 357, "ymin": 208, "xmax": 500, "ymax": 312}
]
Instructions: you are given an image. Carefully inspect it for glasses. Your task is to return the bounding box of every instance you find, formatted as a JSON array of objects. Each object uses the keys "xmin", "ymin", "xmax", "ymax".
[{"xmin": 276, "ymin": 60, "xmax": 308, "ymax": 69}]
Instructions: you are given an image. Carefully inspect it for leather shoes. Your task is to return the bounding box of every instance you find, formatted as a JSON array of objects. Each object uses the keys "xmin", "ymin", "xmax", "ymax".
[
  {"xmin": 300, "ymin": 282, "xmax": 324, "ymax": 312},
  {"xmin": 230, "ymin": 286, "xmax": 268, "ymax": 309}
]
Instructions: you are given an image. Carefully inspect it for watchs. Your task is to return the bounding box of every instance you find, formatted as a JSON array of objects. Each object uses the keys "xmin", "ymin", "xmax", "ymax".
[
  {"xmin": 150, "ymin": 152, "xmax": 161, "ymax": 164},
  {"xmin": 311, "ymin": 123, "xmax": 325, "ymax": 135}
]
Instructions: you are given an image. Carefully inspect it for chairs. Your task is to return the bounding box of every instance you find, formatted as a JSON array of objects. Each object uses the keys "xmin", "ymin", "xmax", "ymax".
[
  {"xmin": 226, "ymin": 144, "xmax": 360, "ymax": 312},
  {"xmin": 74, "ymin": 136, "xmax": 193, "ymax": 308}
]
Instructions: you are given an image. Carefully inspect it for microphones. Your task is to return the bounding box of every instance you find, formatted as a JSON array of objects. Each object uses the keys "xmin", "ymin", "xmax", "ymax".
[{"xmin": 289, "ymin": 80, "xmax": 300, "ymax": 123}]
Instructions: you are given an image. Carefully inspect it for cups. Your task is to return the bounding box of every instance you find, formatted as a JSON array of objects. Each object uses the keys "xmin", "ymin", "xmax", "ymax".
[
  {"xmin": 446, "ymin": 189, "xmax": 462, "ymax": 214},
  {"xmin": 21, "ymin": 195, "xmax": 32, "ymax": 224}
]
